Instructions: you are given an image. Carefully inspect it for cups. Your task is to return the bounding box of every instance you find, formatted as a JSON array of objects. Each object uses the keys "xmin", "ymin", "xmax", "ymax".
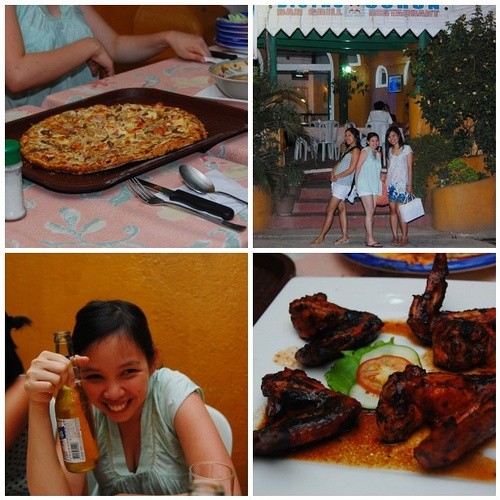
[
  {"xmin": 188, "ymin": 461, "xmax": 234, "ymax": 496},
  {"xmin": 216, "ymin": 12, "xmax": 248, "ymax": 48}
]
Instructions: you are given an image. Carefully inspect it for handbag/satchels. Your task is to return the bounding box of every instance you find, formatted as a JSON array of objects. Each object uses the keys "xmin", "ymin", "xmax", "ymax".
[
  {"xmin": 346, "ymin": 185, "xmax": 358, "ymax": 204},
  {"xmin": 376, "ymin": 174, "xmax": 390, "ymax": 205},
  {"xmin": 398, "ymin": 192, "xmax": 425, "ymax": 224}
]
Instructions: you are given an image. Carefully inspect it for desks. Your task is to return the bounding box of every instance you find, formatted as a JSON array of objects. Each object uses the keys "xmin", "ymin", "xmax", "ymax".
[{"xmin": 302, "ymin": 126, "xmax": 404, "ymax": 146}]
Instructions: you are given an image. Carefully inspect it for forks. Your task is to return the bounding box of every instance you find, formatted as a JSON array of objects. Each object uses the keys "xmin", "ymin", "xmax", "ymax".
[{"xmin": 124, "ymin": 177, "xmax": 246, "ymax": 233}]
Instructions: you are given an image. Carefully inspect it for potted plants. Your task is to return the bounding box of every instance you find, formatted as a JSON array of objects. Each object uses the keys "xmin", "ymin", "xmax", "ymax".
[
  {"xmin": 272, "ymin": 181, "xmax": 295, "ymax": 216},
  {"xmin": 284, "ymin": 163, "xmax": 306, "ymax": 201}
]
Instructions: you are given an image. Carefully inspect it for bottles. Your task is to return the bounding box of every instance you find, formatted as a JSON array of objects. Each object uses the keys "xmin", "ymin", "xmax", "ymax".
[
  {"xmin": 5, "ymin": 139, "xmax": 27, "ymax": 222},
  {"xmin": 54, "ymin": 330, "xmax": 99, "ymax": 473}
]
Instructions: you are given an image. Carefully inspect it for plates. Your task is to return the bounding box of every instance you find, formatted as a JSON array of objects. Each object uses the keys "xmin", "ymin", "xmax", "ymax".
[
  {"xmin": 213, "ymin": 38, "xmax": 247, "ymax": 54},
  {"xmin": 253, "ymin": 276, "xmax": 496, "ymax": 497},
  {"xmin": 341, "ymin": 254, "xmax": 496, "ymax": 274}
]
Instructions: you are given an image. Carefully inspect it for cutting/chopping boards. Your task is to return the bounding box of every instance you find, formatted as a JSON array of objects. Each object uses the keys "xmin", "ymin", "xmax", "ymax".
[{"xmin": 5, "ymin": 88, "xmax": 248, "ymax": 194}]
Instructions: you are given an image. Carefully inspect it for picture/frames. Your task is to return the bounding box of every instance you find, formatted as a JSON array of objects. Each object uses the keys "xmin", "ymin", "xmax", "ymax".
[{"xmin": 388, "ymin": 74, "xmax": 403, "ymax": 92}]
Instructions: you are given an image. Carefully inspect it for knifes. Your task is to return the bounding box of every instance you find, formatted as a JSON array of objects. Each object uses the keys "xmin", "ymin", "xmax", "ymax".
[
  {"xmin": 210, "ymin": 51, "xmax": 244, "ymax": 60},
  {"xmin": 130, "ymin": 178, "xmax": 234, "ymax": 220}
]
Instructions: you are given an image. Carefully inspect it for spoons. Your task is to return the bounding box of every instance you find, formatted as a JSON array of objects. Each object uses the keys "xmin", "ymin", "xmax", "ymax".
[{"xmin": 179, "ymin": 164, "xmax": 249, "ymax": 206}]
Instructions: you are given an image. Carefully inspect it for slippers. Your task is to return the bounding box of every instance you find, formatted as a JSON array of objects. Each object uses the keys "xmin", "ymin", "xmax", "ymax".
[
  {"xmin": 398, "ymin": 239, "xmax": 408, "ymax": 245},
  {"xmin": 391, "ymin": 240, "xmax": 398, "ymax": 246},
  {"xmin": 364, "ymin": 241, "xmax": 383, "ymax": 247}
]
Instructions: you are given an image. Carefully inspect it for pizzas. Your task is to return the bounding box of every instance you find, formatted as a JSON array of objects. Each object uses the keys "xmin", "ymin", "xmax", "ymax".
[{"xmin": 20, "ymin": 102, "xmax": 211, "ymax": 178}]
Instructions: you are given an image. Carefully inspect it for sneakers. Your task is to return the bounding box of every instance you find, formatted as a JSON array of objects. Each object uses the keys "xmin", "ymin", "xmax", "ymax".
[
  {"xmin": 335, "ymin": 238, "xmax": 350, "ymax": 244},
  {"xmin": 310, "ymin": 238, "xmax": 324, "ymax": 247}
]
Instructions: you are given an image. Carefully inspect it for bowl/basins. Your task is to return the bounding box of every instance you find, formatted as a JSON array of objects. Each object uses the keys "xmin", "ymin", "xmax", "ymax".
[{"xmin": 208, "ymin": 58, "xmax": 248, "ymax": 100}]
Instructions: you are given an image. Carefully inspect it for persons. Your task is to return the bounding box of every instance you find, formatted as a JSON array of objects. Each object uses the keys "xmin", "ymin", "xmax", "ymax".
[
  {"xmin": 355, "ymin": 132, "xmax": 384, "ymax": 247},
  {"xmin": 5, "ymin": 311, "xmax": 29, "ymax": 453},
  {"xmin": 311, "ymin": 128, "xmax": 361, "ymax": 246},
  {"xmin": 5, "ymin": 4, "xmax": 213, "ymax": 108},
  {"xmin": 367, "ymin": 101, "xmax": 393, "ymax": 129},
  {"xmin": 25, "ymin": 298, "xmax": 243, "ymax": 495},
  {"xmin": 384, "ymin": 104, "xmax": 397, "ymax": 122},
  {"xmin": 386, "ymin": 126, "xmax": 414, "ymax": 246}
]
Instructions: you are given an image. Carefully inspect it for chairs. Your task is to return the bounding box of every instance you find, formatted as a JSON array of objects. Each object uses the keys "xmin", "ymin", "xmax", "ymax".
[
  {"xmin": 365, "ymin": 121, "xmax": 390, "ymax": 166},
  {"xmin": 344, "ymin": 121, "xmax": 356, "ymax": 128},
  {"xmin": 309, "ymin": 119, "xmax": 322, "ymax": 126},
  {"xmin": 91, "ymin": 402, "xmax": 233, "ymax": 495},
  {"xmin": 293, "ymin": 134, "xmax": 314, "ymax": 161}
]
